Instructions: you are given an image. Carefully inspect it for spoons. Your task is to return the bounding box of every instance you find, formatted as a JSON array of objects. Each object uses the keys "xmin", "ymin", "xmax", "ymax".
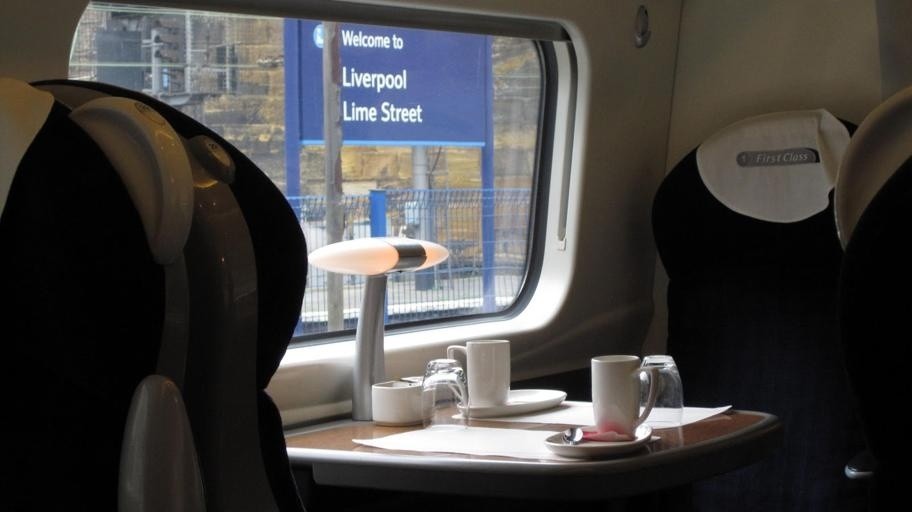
[{"xmin": 561, "ymin": 426, "xmax": 584, "ymax": 447}]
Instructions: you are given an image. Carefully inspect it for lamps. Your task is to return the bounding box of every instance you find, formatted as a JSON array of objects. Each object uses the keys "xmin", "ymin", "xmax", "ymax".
[{"xmin": 307, "ymin": 237, "xmax": 449, "ymax": 421}]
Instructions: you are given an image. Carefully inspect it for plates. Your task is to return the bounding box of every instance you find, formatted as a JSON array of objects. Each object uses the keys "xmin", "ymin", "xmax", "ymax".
[
  {"xmin": 543, "ymin": 422, "xmax": 654, "ymax": 458},
  {"xmin": 456, "ymin": 389, "xmax": 567, "ymax": 417}
]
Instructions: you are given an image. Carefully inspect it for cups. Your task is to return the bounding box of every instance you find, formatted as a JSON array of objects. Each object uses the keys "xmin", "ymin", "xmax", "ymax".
[
  {"xmin": 421, "ymin": 358, "xmax": 470, "ymax": 434},
  {"xmin": 590, "ymin": 353, "xmax": 659, "ymax": 442},
  {"xmin": 641, "ymin": 355, "xmax": 684, "ymax": 424},
  {"xmin": 446, "ymin": 339, "xmax": 511, "ymax": 406},
  {"xmin": 372, "ymin": 379, "xmax": 435, "ymax": 427}
]
